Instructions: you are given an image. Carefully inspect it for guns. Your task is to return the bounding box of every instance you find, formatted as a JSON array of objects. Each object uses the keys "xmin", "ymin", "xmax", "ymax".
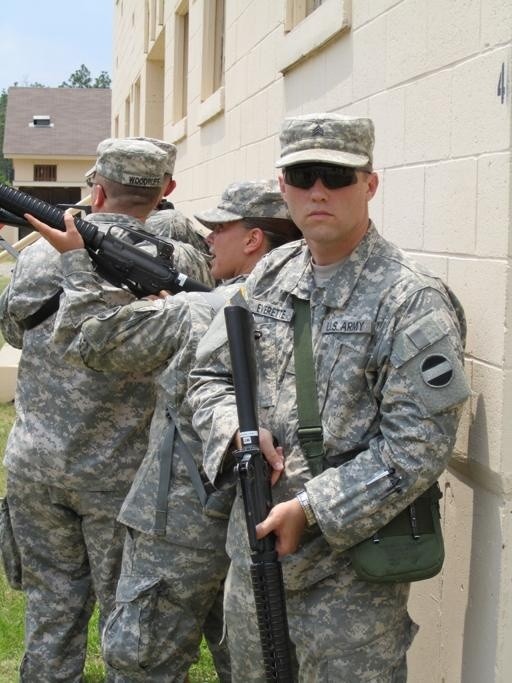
[
  {"xmin": 224, "ymin": 306, "xmax": 295, "ymax": 679},
  {"xmin": 0, "ymin": 180, "xmax": 212, "ymax": 296}
]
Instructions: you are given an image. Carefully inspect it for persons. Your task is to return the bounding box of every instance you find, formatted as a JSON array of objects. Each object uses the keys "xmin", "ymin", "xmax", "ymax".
[
  {"xmin": 119, "ymin": 137, "xmax": 211, "ymax": 255},
  {"xmin": 189, "ymin": 113, "xmax": 472, "ymax": 682},
  {"xmin": 25, "ymin": 180, "xmax": 307, "ymax": 682},
  {"xmin": 1, "ymin": 138, "xmax": 218, "ymax": 683}
]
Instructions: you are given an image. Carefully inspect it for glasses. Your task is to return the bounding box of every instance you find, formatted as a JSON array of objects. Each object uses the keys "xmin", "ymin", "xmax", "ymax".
[
  {"xmin": 87, "ymin": 178, "xmax": 107, "ymax": 199},
  {"xmin": 284, "ymin": 162, "xmax": 358, "ymax": 189}
]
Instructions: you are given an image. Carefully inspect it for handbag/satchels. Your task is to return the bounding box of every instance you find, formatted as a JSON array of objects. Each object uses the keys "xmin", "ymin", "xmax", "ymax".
[{"xmin": 321, "ymin": 448, "xmax": 444, "ymax": 584}]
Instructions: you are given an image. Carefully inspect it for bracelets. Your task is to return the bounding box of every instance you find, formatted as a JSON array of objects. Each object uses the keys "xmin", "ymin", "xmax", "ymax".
[{"xmin": 296, "ymin": 489, "xmax": 317, "ymax": 528}]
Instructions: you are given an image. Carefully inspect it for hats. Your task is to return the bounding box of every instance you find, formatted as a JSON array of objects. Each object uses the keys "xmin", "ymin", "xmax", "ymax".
[
  {"xmin": 85, "ymin": 138, "xmax": 168, "ymax": 188},
  {"xmin": 120, "ymin": 136, "xmax": 176, "ymax": 175},
  {"xmin": 194, "ymin": 177, "xmax": 292, "ymax": 231},
  {"xmin": 275, "ymin": 114, "xmax": 375, "ymax": 172}
]
